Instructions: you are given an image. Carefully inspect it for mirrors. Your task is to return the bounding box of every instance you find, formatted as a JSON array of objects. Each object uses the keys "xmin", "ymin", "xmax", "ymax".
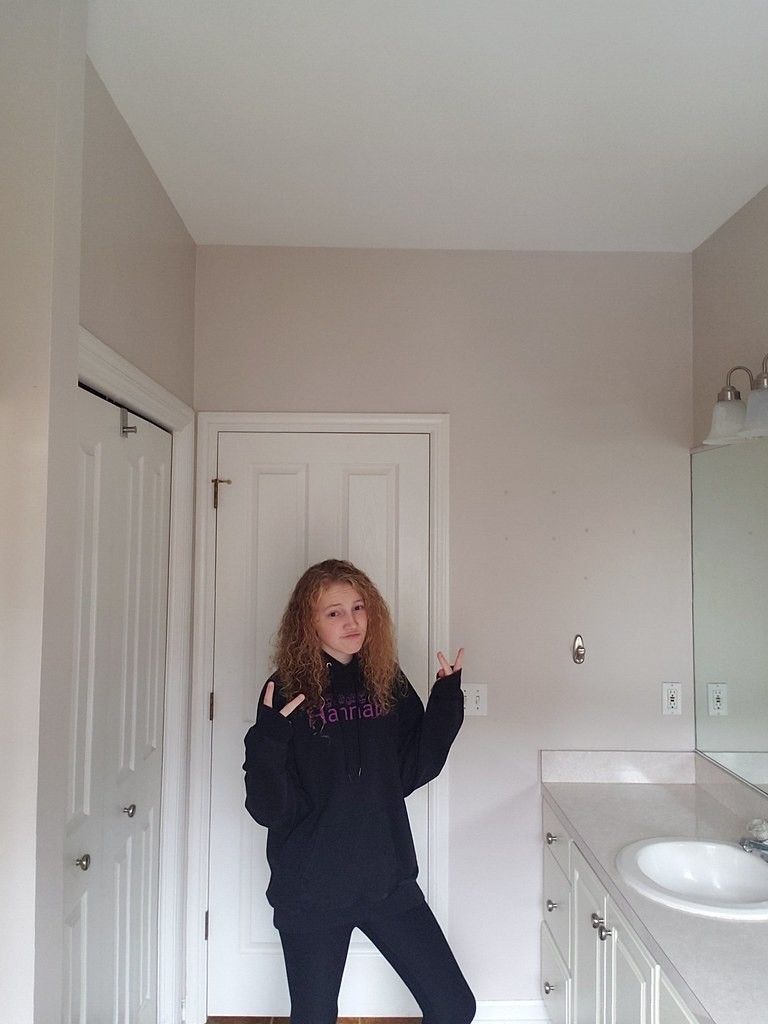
[{"xmin": 690, "ymin": 436, "xmax": 768, "ymax": 797}]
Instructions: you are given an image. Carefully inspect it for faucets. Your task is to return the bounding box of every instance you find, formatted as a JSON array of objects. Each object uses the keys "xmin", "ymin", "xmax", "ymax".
[{"xmin": 737, "ymin": 816, "xmax": 768, "ymax": 862}]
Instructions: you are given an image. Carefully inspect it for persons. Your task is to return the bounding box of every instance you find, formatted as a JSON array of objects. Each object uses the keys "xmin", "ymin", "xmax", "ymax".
[{"xmin": 240, "ymin": 557, "xmax": 476, "ymax": 1024}]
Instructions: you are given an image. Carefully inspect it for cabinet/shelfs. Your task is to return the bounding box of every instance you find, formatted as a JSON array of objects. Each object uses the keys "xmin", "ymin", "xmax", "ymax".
[{"xmin": 540, "ymin": 788, "xmax": 715, "ymax": 1024}]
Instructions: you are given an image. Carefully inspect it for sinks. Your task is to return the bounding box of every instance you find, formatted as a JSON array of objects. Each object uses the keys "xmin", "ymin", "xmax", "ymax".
[{"xmin": 613, "ymin": 834, "xmax": 768, "ymax": 925}]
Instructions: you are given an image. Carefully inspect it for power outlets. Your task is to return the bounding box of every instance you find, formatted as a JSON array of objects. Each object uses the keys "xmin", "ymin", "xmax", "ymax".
[
  {"xmin": 460, "ymin": 683, "xmax": 488, "ymax": 715},
  {"xmin": 707, "ymin": 683, "xmax": 728, "ymax": 717},
  {"xmin": 661, "ymin": 682, "xmax": 682, "ymax": 716}
]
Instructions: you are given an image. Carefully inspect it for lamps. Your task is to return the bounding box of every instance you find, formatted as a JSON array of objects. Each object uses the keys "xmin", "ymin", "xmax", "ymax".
[
  {"xmin": 739, "ymin": 353, "xmax": 768, "ymax": 438},
  {"xmin": 702, "ymin": 366, "xmax": 755, "ymax": 447}
]
